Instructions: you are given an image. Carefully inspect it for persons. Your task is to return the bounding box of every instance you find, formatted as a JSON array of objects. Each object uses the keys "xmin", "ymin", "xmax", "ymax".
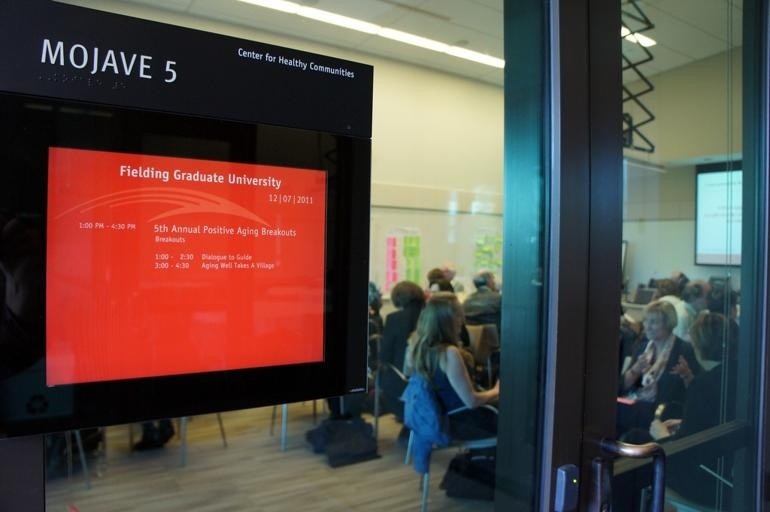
[
  {"xmin": 65, "ymin": 427, "xmax": 106, "ymax": 455},
  {"xmin": 129, "ymin": 418, "xmax": 176, "ymax": 452},
  {"xmin": 365, "ymin": 264, "xmax": 503, "ymax": 467},
  {"xmin": 614, "ymin": 271, "xmax": 742, "ymax": 440}
]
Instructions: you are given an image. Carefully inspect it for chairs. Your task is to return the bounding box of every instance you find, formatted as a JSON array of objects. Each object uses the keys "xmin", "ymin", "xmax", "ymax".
[{"xmin": 367, "ymin": 278, "xmax": 741, "ymax": 508}]
